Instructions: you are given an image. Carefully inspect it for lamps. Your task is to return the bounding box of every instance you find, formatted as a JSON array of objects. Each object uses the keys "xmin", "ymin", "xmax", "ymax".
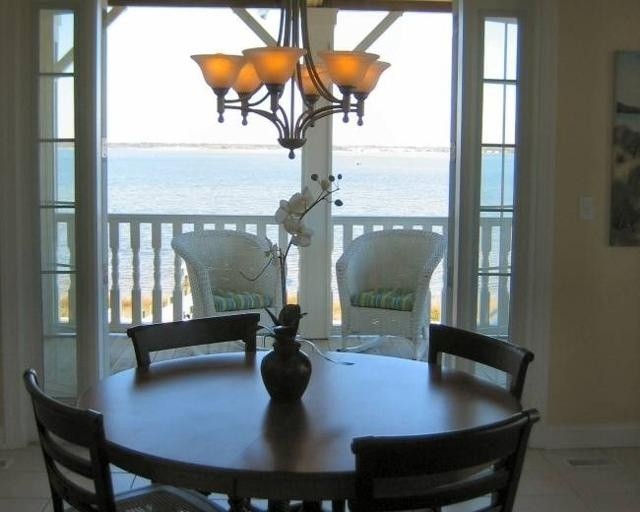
[{"xmin": 191, "ymin": 0, "xmax": 392, "ymax": 159}]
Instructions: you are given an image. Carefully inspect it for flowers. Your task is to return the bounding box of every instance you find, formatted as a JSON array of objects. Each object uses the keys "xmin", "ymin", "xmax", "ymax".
[{"xmin": 237, "ymin": 172, "xmax": 343, "ymax": 342}]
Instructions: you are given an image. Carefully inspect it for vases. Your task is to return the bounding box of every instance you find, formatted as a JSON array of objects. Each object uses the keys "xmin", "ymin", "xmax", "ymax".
[{"xmin": 261, "ymin": 340, "xmax": 313, "ymax": 404}]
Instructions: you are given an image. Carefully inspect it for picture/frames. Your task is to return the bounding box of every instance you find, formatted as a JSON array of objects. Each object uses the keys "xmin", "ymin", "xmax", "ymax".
[{"xmin": 609, "ymin": 49, "xmax": 640, "ymax": 248}]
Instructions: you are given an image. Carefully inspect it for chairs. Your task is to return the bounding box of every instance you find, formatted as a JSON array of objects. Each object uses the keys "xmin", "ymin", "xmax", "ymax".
[
  {"xmin": 127, "ymin": 315, "xmax": 259, "ymax": 367},
  {"xmin": 172, "ymin": 231, "xmax": 286, "ymax": 357},
  {"xmin": 429, "ymin": 325, "xmax": 535, "ymax": 398},
  {"xmin": 334, "ymin": 229, "xmax": 449, "ymax": 358},
  {"xmin": 353, "ymin": 408, "xmax": 539, "ymax": 511},
  {"xmin": 21, "ymin": 368, "xmax": 224, "ymax": 512}
]
